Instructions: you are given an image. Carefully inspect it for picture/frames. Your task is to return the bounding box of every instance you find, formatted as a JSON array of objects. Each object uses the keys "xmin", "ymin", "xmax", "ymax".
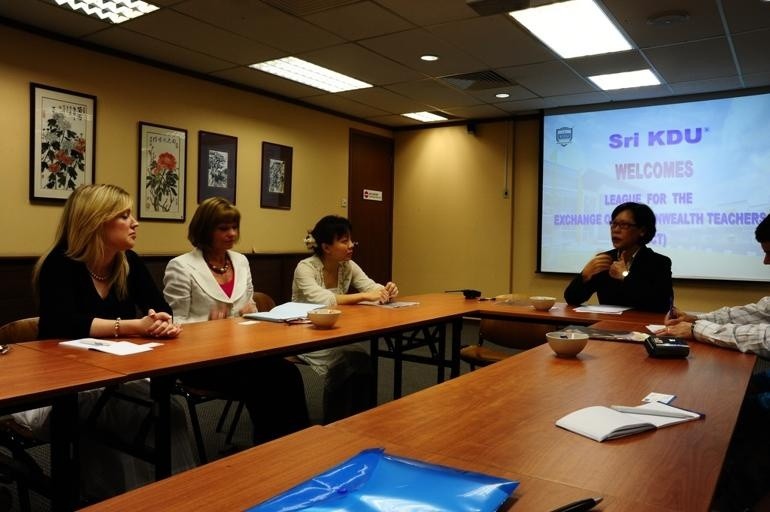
[
  {"xmin": 30, "ymin": 82, "xmax": 97, "ymax": 206},
  {"xmin": 197, "ymin": 129, "xmax": 239, "ymax": 207},
  {"xmin": 137, "ymin": 120, "xmax": 187, "ymax": 224},
  {"xmin": 260, "ymin": 140, "xmax": 293, "ymax": 213}
]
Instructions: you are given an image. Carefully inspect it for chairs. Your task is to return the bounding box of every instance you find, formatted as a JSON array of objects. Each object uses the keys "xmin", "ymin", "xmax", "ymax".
[
  {"xmin": 459, "ymin": 288, "xmax": 556, "ymax": 368},
  {"xmin": 251, "ymin": 289, "xmax": 311, "ymax": 366},
  {"xmin": 174, "ymin": 379, "xmax": 245, "ymax": 464},
  {"xmin": 0, "ymin": 316, "xmax": 111, "ymax": 511}
]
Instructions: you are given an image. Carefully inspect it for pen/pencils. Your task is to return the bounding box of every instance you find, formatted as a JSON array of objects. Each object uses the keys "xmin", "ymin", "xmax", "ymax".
[
  {"xmin": 478, "ymin": 297, "xmax": 497, "ymax": 302},
  {"xmin": 670, "ymin": 301, "xmax": 674, "ymax": 318},
  {"xmin": 549, "ymin": 497, "xmax": 602, "ymax": 512}
]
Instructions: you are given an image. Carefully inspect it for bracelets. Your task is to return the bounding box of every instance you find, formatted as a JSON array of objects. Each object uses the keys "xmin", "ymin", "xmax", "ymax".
[
  {"xmin": 691, "ymin": 321, "xmax": 696, "ymax": 337},
  {"xmin": 114, "ymin": 317, "xmax": 121, "ymax": 338}
]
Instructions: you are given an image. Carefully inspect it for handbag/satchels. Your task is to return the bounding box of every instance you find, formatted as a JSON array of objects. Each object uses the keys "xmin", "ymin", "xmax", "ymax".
[{"xmin": 9, "ymin": 405, "xmax": 52, "ymax": 431}]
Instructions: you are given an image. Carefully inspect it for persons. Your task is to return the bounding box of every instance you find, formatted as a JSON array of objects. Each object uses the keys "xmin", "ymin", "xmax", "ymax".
[
  {"xmin": 653, "ymin": 212, "xmax": 770, "ymax": 415},
  {"xmin": 163, "ymin": 196, "xmax": 311, "ymax": 448},
  {"xmin": 291, "ymin": 215, "xmax": 399, "ymax": 426},
  {"xmin": 564, "ymin": 202, "xmax": 674, "ymax": 315},
  {"xmin": 33, "ymin": 184, "xmax": 202, "ymax": 496}
]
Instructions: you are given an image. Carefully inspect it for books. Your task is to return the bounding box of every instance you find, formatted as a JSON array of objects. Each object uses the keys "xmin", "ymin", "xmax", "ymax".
[
  {"xmin": 555, "ymin": 402, "xmax": 704, "ymax": 443},
  {"xmin": 59, "ymin": 338, "xmax": 153, "ymax": 356},
  {"xmin": 242, "ymin": 302, "xmax": 328, "ymax": 322}
]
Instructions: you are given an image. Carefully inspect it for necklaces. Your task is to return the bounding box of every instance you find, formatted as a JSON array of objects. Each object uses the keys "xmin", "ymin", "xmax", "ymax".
[
  {"xmin": 88, "ymin": 266, "xmax": 111, "ymax": 281},
  {"xmin": 204, "ymin": 253, "xmax": 230, "ymax": 274}
]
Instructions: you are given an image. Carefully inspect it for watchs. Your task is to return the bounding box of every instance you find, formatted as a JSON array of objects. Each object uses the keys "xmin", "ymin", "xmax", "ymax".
[{"xmin": 622, "ymin": 271, "xmax": 630, "ymax": 280}]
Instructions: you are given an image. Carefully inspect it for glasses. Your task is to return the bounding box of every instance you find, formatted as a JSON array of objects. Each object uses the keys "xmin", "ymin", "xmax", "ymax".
[
  {"xmin": 610, "ymin": 222, "xmax": 636, "ymax": 229},
  {"xmin": 285, "ymin": 317, "xmax": 312, "ymax": 324}
]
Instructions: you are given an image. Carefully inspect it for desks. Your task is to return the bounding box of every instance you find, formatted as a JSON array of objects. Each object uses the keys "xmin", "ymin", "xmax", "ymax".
[
  {"xmin": 82, "ymin": 421, "xmax": 691, "ymax": 510},
  {"xmin": 0, "ymin": 292, "xmax": 125, "ymax": 511},
  {"xmin": 18, "ymin": 291, "xmax": 483, "ymax": 480},
  {"xmin": 323, "ymin": 323, "xmax": 758, "ymax": 511},
  {"xmin": 473, "ymin": 292, "xmax": 678, "ymax": 323}
]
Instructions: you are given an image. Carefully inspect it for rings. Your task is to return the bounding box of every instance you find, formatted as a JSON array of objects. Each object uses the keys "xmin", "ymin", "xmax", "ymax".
[{"xmin": 667, "ymin": 328, "xmax": 670, "ymax": 334}]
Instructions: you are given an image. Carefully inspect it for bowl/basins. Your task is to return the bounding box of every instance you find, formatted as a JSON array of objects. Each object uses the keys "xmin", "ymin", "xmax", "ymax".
[
  {"xmin": 529, "ymin": 295, "xmax": 557, "ymax": 312},
  {"xmin": 546, "ymin": 331, "xmax": 590, "ymax": 357},
  {"xmin": 308, "ymin": 309, "xmax": 342, "ymax": 328}
]
